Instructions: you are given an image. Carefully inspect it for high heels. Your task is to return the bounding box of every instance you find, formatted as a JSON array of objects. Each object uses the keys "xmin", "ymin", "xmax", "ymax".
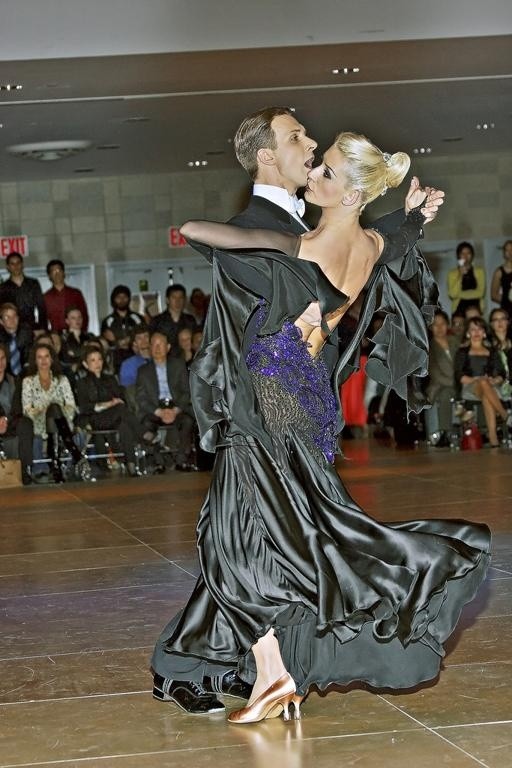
[
  {"xmin": 486, "ymin": 432, "xmax": 501, "ymax": 449},
  {"xmin": 265, "ymin": 687, "xmax": 309, "ymax": 720},
  {"xmin": 504, "ymin": 414, "xmax": 512, "ymax": 428},
  {"xmin": 227, "ymin": 673, "xmax": 297, "ymax": 723}
]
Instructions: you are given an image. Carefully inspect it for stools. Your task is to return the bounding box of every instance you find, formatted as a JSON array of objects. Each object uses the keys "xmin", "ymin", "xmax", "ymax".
[{"xmin": 463, "ymin": 399, "xmax": 508, "ymax": 446}]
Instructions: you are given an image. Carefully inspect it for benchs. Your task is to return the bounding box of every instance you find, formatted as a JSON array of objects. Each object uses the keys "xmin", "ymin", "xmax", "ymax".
[{"xmin": 21, "ymin": 423, "xmax": 196, "ymax": 484}]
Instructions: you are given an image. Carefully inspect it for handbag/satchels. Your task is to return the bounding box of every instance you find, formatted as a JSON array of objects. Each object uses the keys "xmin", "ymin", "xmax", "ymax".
[
  {"xmin": 462, "ymin": 422, "xmax": 482, "ymax": 450},
  {"xmin": 1, "ymin": 455, "xmax": 23, "ymax": 490}
]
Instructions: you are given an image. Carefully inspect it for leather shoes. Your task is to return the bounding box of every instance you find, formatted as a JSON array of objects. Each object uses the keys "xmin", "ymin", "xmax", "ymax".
[
  {"xmin": 153, "ymin": 673, "xmax": 226, "ymax": 715},
  {"xmin": 204, "ymin": 670, "xmax": 254, "ymax": 700},
  {"xmin": 177, "ymin": 463, "xmax": 200, "ymax": 472}
]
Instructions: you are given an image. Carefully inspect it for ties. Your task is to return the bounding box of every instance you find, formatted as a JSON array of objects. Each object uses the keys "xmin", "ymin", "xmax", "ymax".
[{"xmin": 9, "ymin": 335, "xmax": 22, "ymax": 375}]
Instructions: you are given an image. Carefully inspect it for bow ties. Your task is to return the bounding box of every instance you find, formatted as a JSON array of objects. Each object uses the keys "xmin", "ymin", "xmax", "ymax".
[{"xmin": 290, "ymin": 197, "xmax": 305, "ymax": 218}]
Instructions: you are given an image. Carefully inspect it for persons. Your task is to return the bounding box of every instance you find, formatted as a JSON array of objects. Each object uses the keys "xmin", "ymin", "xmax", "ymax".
[
  {"xmin": 72, "ymin": 338, "xmax": 110, "ymax": 405},
  {"xmin": 58, "ymin": 304, "xmax": 97, "ymax": 373},
  {"xmin": 151, "ymin": 106, "xmax": 447, "ymax": 715},
  {"xmin": 448, "ymin": 311, "xmax": 466, "ymax": 338},
  {"xmin": 74, "ymin": 340, "xmax": 117, "ymax": 380},
  {"xmin": 101, "ymin": 284, "xmax": 147, "ymax": 375},
  {"xmin": 20, "ymin": 333, "xmax": 55, "ymax": 377},
  {"xmin": 1, "ymin": 302, "xmax": 35, "ymax": 379},
  {"xmin": 177, "ymin": 326, "xmax": 195, "ymax": 367},
  {"xmin": 113, "ymin": 330, "xmax": 136, "ymax": 374},
  {"xmin": 118, "ymin": 328, "xmax": 151, "ymax": 386},
  {"xmin": 428, "ymin": 310, "xmax": 462, "ymax": 449},
  {"xmin": 32, "ymin": 322, "xmax": 46, "ymax": 340},
  {"xmin": 466, "ymin": 306, "xmax": 482, "ymax": 319},
  {"xmin": 490, "ymin": 240, "xmax": 512, "ymax": 315},
  {"xmin": 488, "ymin": 308, "xmax": 512, "ymax": 412},
  {"xmin": 164, "ymin": 128, "xmax": 489, "ymax": 728},
  {"xmin": 453, "ymin": 316, "xmax": 512, "ymax": 448},
  {"xmin": 20, "ymin": 343, "xmax": 88, "ymax": 485},
  {"xmin": 134, "ymin": 329, "xmax": 194, "ymax": 475},
  {"xmin": 74, "ymin": 344, "xmax": 161, "ymax": 479},
  {"xmin": 149, "ymin": 283, "xmax": 198, "ymax": 358},
  {"xmin": 361, "ymin": 318, "xmax": 384, "ymax": 350},
  {"xmin": 192, "ymin": 329, "xmax": 204, "ymax": 354},
  {"xmin": 447, "ymin": 240, "xmax": 486, "ymax": 317},
  {"xmin": 43, "ymin": 259, "xmax": 89, "ymax": 332},
  {"xmin": 0, "ymin": 251, "xmax": 48, "ymax": 331},
  {"xmin": 0, "ymin": 343, "xmax": 39, "ymax": 485},
  {"xmin": 190, "ymin": 288, "xmax": 209, "ymax": 323},
  {"xmin": 49, "ymin": 333, "xmax": 76, "ymax": 392}
]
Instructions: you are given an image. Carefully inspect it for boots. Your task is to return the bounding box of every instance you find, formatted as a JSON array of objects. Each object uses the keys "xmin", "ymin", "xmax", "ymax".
[
  {"xmin": 47, "ymin": 432, "xmax": 65, "ymax": 484},
  {"xmin": 54, "ymin": 416, "xmax": 84, "ymax": 460}
]
